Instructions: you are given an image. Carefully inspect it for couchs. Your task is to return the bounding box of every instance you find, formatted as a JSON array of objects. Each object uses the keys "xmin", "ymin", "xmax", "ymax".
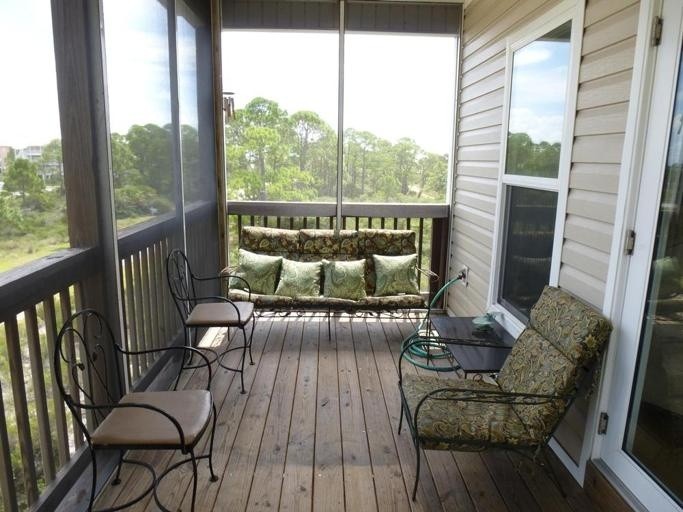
[{"xmin": 218, "ymin": 226, "xmax": 440, "ymax": 345}]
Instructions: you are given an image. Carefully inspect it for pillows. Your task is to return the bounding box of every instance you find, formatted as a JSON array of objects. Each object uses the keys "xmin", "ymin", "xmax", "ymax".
[{"xmin": 228, "ymin": 247, "xmax": 420, "ymax": 301}]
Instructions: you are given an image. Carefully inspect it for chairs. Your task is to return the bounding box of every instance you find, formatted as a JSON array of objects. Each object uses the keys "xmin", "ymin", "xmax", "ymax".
[
  {"xmin": 166, "ymin": 248, "xmax": 255, "ymax": 394},
  {"xmin": 397, "ymin": 285, "xmax": 612, "ymax": 501},
  {"xmin": 53, "ymin": 308, "xmax": 217, "ymax": 512}
]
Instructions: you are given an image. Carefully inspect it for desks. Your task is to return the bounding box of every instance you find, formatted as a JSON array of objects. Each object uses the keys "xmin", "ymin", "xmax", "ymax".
[{"xmin": 426, "ymin": 315, "xmax": 517, "ymax": 380}]
